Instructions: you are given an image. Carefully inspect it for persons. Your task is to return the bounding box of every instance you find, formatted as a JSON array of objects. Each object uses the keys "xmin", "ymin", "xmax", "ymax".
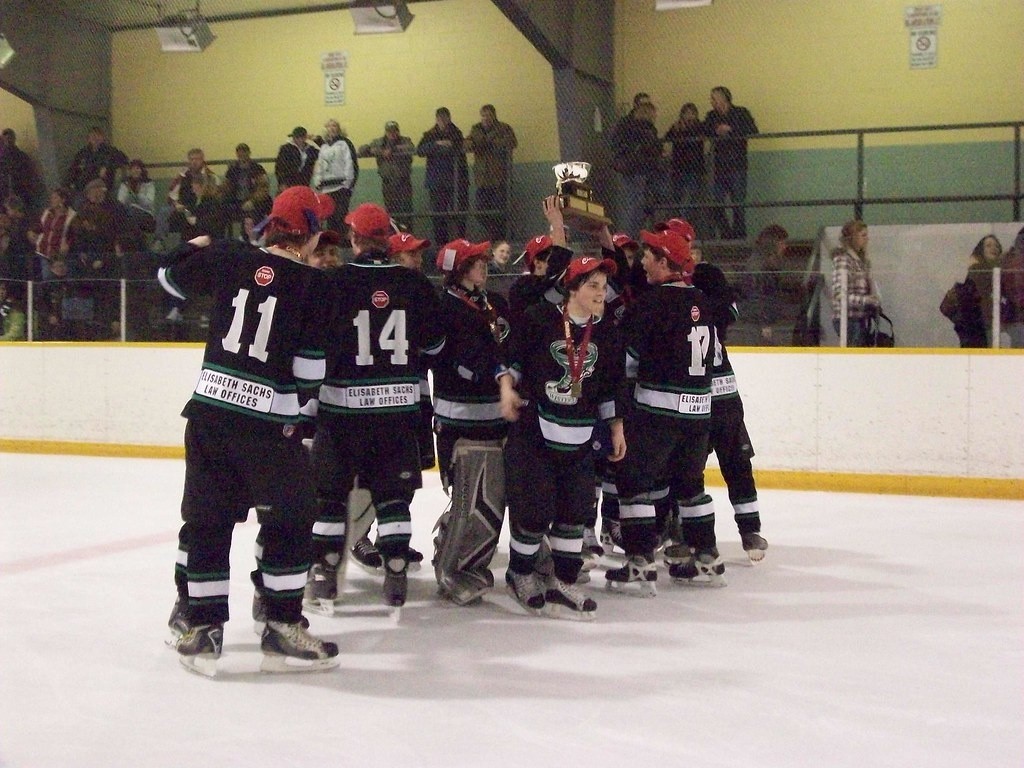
[
  {"xmin": 461, "ymin": 102, "xmax": 518, "ymax": 240},
  {"xmin": 418, "ymin": 107, "xmax": 470, "ymax": 246},
  {"xmin": 275, "ymin": 126, "xmax": 328, "ymax": 189},
  {"xmin": 356, "ymin": 121, "xmax": 415, "ymax": 235},
  {"xmin": 599, "ymin": 85, "xmax": 761, "ymax": 242},
  {"xmin": 945, "ymin": 238, "xmax": 1003, "ymax": 350},
  {"xmin": 728, "ymin": 224, "xmax": 810, "ymax": 343},
  {"xmin": 307, "ymin": 117, "xmax": 360, "ymax": 235},
  {"xmin": 827, "ymin": 221, "xmax": 884, "ymax": 347},
  {"xmin": 1, "ymin": 106, "xmax": 273, "ymax": 343},
  {"xmin": 1000, "ymin": 228, "xmax": 1023, "ymax": 353},
  {"xmin": 157, "ymin": 183, "xmax": 768, "ymax": 679}
]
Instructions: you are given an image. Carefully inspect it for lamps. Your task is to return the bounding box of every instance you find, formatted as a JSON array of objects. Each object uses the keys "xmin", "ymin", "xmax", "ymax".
[
  {"xmin": 351, "ymin": 0, "xmax": 416, "ymax": 36},
  {"xmin": 153, "ymin": 11, "xmax": 219, "ymax": 53}
]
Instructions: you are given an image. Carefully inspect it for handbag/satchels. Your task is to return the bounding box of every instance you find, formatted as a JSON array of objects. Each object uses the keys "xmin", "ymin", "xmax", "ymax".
[
  {"xmin": 937, "ymin": 277, "xmax": 982, "ymax": 329},
  {"xmin": 865, "ymin": 311, "xmax": 896, "ymax": 348}
]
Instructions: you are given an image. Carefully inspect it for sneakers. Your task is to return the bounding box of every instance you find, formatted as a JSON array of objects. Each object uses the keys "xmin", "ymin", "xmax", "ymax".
[
  {"xmin": 604, "ymin": 504, "xmax": 724, "ymax": 594},
  {"xmin": 373, "ymin": 527, "xmax": 423, "ymax": 572},
  {"xmin": 739, "ymin": 517, "xmax": 769, "ymax": 565},
  {"xmin": 164, "ymin": 570, "xmax": 309, "ymax": 651},
  {"xmin": 503, "ymin": 543, "xmax": 545, "ymax": 618},
  {"xmin": 176, "ymin": 625, "xmax": 339, "ymax": 676},
  {"xmin": 377, "ymin": 536, "xmax": 409, "ymax": 625},
  {"xmin": 544, "ymin": 557, "xmax": 596, "ymax": 619},
  {"xmin": 580, "ymin": 524, "xmax": 604, "ymax": 554},
  {"xmin": 350, "ymin": 534, "xmax": 386, "ymax": 577},
  {"xmin": 302, "ymin": 551, "xmax": 343, "ymax": 619}
]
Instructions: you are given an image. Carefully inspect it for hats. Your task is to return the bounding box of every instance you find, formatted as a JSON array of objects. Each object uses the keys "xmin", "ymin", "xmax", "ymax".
[
  {"xmin": 288, "ymin": 127, "xmax": 307, "ymax": 137},
  {"xmin": 384, "ymin": 121, "xmax": 399, "ymax": 131},
  {"xmin": 262, "ymin": 187, "xmax": 703, "ymax": 287}
]
